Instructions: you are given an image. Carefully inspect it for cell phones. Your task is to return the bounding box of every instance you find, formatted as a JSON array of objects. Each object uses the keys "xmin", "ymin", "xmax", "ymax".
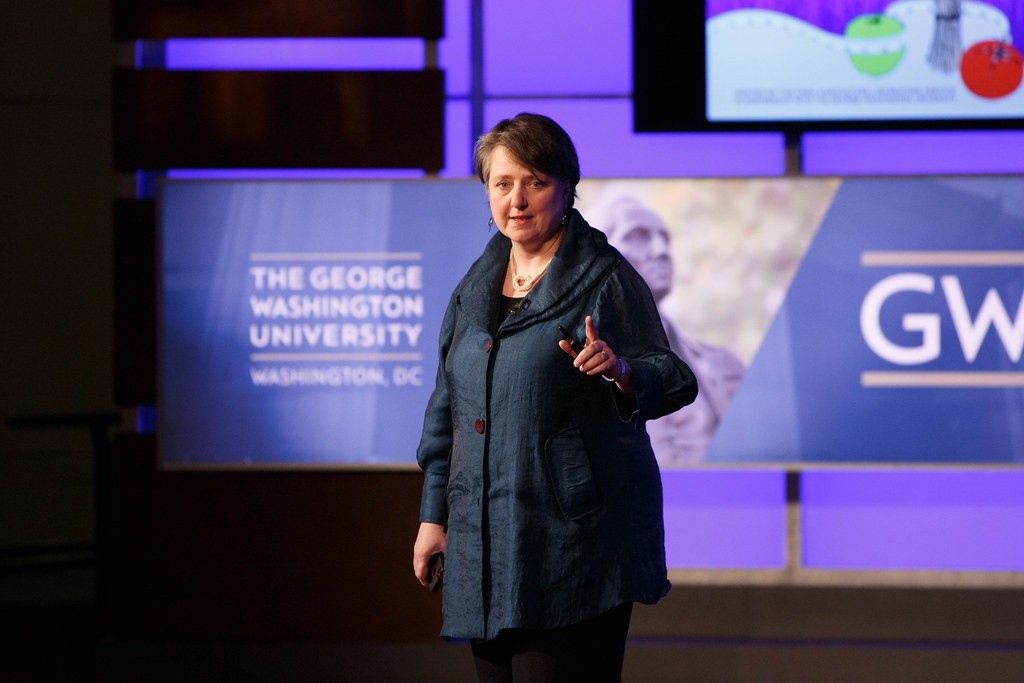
[{"xmin": 427, "ymin": 552, "xmax": 446, "ymax": 592}]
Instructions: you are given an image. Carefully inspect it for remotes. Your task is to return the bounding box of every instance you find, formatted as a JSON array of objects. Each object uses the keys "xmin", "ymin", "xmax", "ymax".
[{"xmin": 559, "ymin": 325, "xmax": 584, "ymax": 356}]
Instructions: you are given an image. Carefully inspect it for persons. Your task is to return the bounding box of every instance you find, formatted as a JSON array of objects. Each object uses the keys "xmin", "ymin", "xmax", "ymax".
[
  {"xmin": 412, "ymin": 113, "xmax": 699, "ymax": 683},
  {"xmin": 586, "ymin": 199, "xmax": 747, "ymax": 461}
]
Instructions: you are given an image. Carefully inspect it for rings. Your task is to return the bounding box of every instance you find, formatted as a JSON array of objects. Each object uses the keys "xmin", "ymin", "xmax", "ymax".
[{"xmin": 602, "ymin": 351, "xmax": 608, "ymax": 359}]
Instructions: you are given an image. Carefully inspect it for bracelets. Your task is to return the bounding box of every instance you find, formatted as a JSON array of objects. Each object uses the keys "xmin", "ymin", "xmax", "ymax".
[{"xmin": 602, "ymin": 355, "xmax": 625, "ymax": 381}]
